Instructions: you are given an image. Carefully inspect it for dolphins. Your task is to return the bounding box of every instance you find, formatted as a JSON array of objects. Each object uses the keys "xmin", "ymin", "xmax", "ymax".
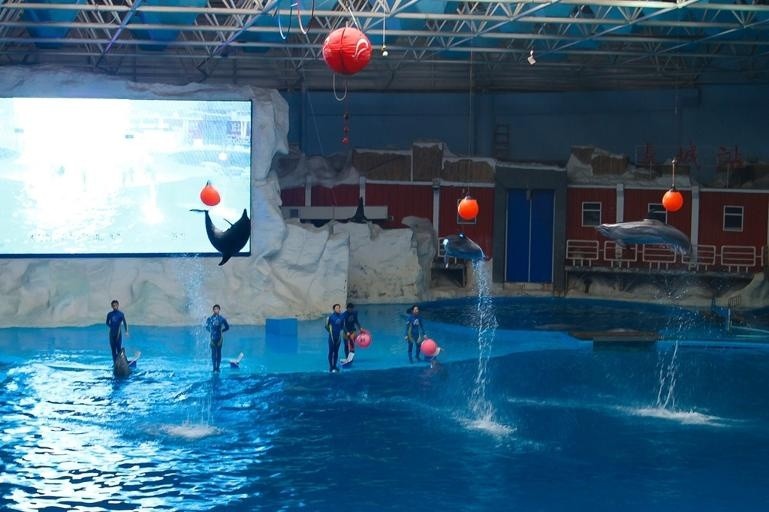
[
  {"xmin": 595, "ymin": 218, "xmax": 691, "ymax": 257},
  {"xmin": 431, "ymin": 359, "xmax": 438, "ymax": 366},
  {"xmin": 228, "ymin": 359, "xmax": 239, "ymax": 369},
  {"xmin": 189, "ymin": 208, "xmax": 251, "ymax": 266},
  {"xmin": 443, "ymin": 232, "xmax": 486, "ymax": 270},
  {"xmin": 113, "ymin": 348, "xmax": 130, "ymax": 379}
]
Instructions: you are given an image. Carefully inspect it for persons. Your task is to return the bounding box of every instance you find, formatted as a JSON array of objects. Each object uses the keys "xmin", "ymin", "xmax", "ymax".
[
  {"xmin": 106, "ymin": 299, "xmax": 129, "ymax": 367},
  {"xmin": 404, "ymin": 304, "xmax": 427, "ymax": 364},
  {"xmin": 342, "ymin": 302, "xmax": 364, "ymax": 359},
  {"xmin": 324, "ymin": 304, "xmax": 350, "ymax": 373},
  {"xmin": 206, "ymin": 304, "xmax": 229, "ymax": 373}
]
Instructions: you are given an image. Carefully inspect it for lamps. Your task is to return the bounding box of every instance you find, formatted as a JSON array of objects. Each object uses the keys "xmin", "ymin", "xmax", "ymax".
[
  {"xmin": 456, "ymin": 27, "xmax": 481, "ymax": 220},
  {"xmin": 660, "ymin": 48, "xmax": 685, "ymax": 213},
  {"xmin": 200, "ymin": 54, "xmax": 221, "ymax": 207}
]
